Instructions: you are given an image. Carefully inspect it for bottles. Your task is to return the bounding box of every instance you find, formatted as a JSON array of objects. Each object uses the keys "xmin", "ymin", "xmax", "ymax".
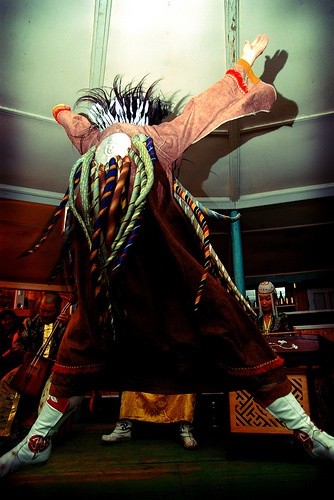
[
  {"xmin": 290, "ymin": 293, "xmax": 294, "ymax": 305},
  {"xmin": 254, "ymin": 301, "xmax": 258, "ymax": 307},
  {"xmin": 279, "ymin": 291, "xmax": 284, "ymax": 305},
  {"xmin": 285, "ymin": 293, "xmax": 289, "ymax": 305}
]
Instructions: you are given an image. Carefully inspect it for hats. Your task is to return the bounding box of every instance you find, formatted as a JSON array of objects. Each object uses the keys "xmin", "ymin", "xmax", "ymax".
[{"xmin": 257, "ymin": 281, "xmax": 275, "ymax": 294}]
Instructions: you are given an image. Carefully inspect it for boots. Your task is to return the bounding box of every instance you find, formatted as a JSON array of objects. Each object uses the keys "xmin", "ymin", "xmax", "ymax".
[
  {"xmin": 102, "ymin": 420, "xmax": 132, "ymax": 441},
  {"xmin": 0, "ymin": 394, "xmax": 80, "ymax": 479},
  {"xmin": 253, "ymin": 376, "xmax": 334, "ymax": 460},
  {"xmin": 177, "ymin": 421, "xmax": 197, "ymax": 447}
]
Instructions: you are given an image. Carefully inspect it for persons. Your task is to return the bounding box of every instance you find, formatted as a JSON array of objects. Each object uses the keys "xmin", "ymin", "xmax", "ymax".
[
  {"xmin": 257, "ymin": 281, "xmax": 293, "ymax": 337},
  {"xmin": 0, "ymin": 33, "xmax": 334, "ymax": 477},
  {"xmin": 100, "ymin": 390, "xmax": 198, "ymax": 448},
  {"xmin": 0, "ymin": 292, "xmax": 71, "ymax": 449}
]
